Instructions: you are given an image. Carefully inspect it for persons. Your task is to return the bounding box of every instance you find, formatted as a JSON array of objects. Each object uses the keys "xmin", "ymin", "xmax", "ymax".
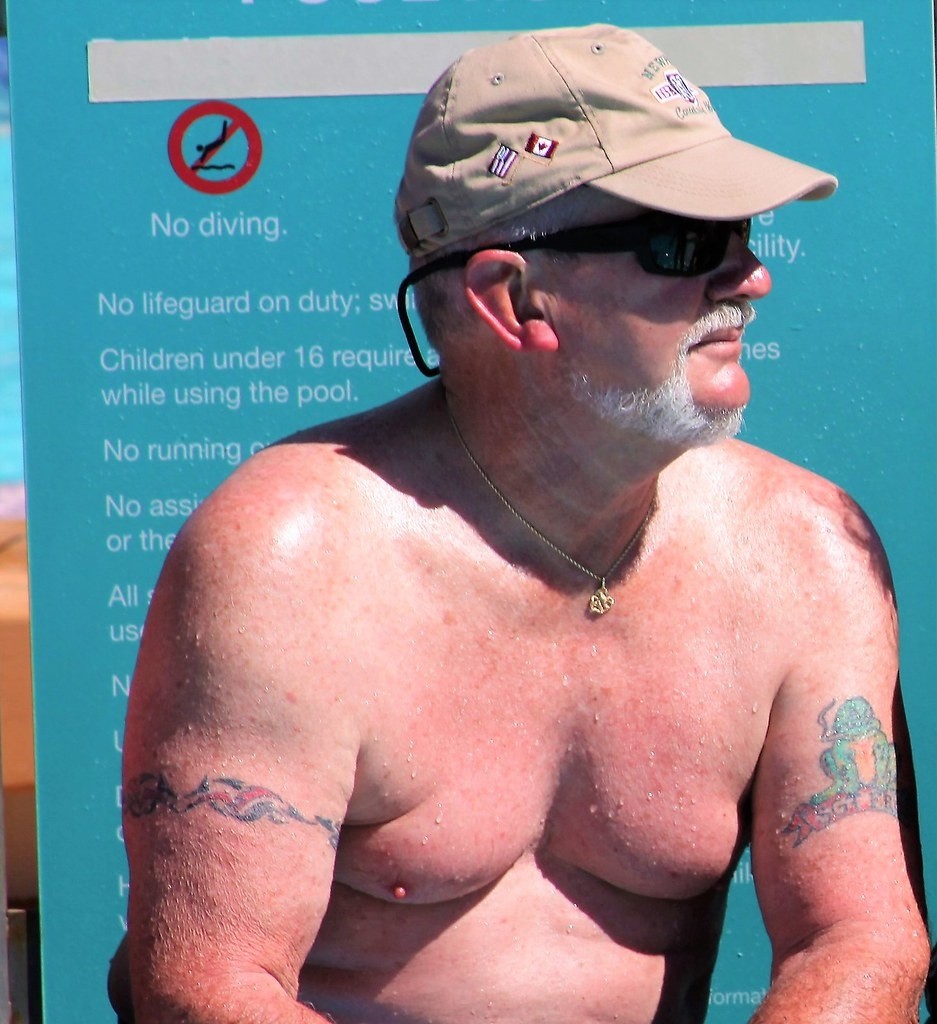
[{"xmin": 104, "ymin": 22, "xmax": 932, "ymax": 1024}]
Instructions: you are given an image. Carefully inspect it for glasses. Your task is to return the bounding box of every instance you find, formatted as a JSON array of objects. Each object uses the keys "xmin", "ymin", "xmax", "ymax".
[{"xmin": 398, "ymin": 209, "xmax": 751, "ymax": 378}]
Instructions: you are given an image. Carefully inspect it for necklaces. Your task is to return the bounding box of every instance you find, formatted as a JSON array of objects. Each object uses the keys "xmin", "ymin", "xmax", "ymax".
[{"xmin": 442, "ymin": 387, "xmax": 663, "ymax": 619}]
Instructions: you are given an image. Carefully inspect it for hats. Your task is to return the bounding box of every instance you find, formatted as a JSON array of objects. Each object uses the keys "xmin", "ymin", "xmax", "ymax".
[{"xmin": 395, "ymin": 27, "xmax": 839, "ymax": 258}]
